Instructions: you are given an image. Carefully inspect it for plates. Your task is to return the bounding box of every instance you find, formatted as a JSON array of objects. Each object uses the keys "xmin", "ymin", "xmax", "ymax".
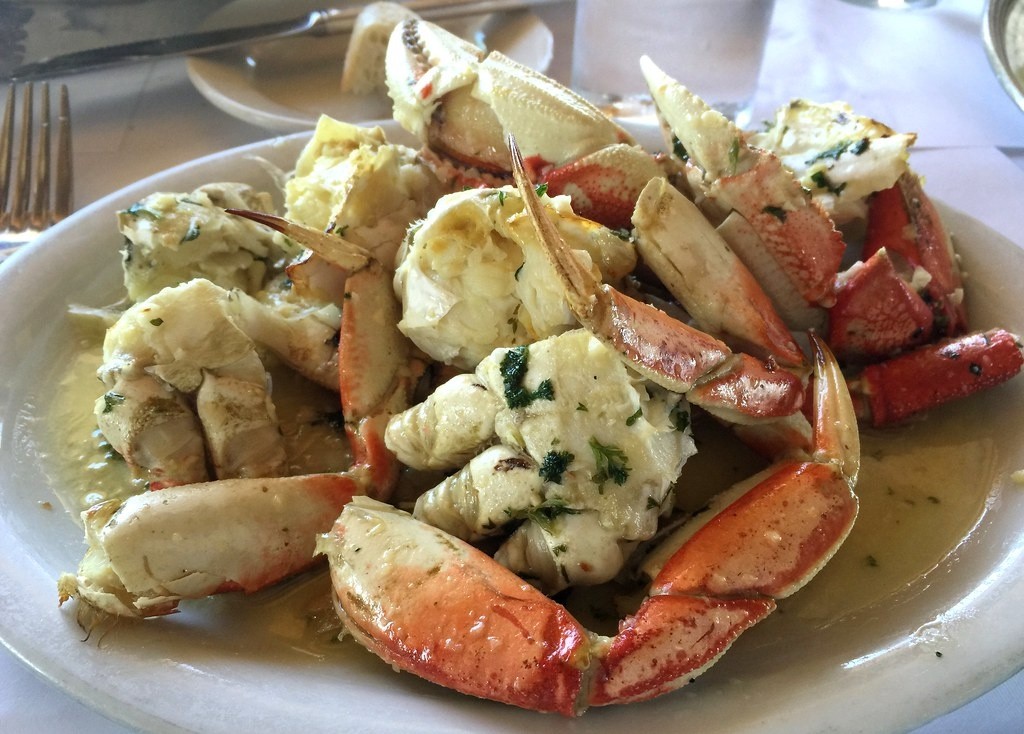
[
  {"xmin": 983, "ymin": 0, "xmax": 1024, "ymax": 113},
  {"xmin": 1, "ymin": 113, "xmax": 1024, "ymax": 734},
  {"xmin": 185, "ymin": 0, "xmax": 553, "ymax": 130}
]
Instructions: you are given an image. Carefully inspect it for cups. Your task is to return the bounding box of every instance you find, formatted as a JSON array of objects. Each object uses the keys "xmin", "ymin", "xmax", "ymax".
[{"xmin": 575, "ymin": 0, "xmax": 773, "ymax": 127}]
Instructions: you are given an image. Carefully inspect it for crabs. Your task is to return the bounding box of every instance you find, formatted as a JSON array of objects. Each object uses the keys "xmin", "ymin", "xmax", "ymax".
[{"xmin": 55, "ymin": 17, "xmax": 1024, "ymax": 717}]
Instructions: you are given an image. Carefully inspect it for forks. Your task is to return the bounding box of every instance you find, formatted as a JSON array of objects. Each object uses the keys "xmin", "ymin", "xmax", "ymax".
[{"xmin": 0, "ymin": 78, "xmax": 71, "ymax": 269}]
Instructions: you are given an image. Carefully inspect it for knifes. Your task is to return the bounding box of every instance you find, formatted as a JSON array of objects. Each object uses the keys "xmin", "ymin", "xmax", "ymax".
[{"xmin": 0, "ymin": 0, "xmax": 542, "ymax": 86}]
